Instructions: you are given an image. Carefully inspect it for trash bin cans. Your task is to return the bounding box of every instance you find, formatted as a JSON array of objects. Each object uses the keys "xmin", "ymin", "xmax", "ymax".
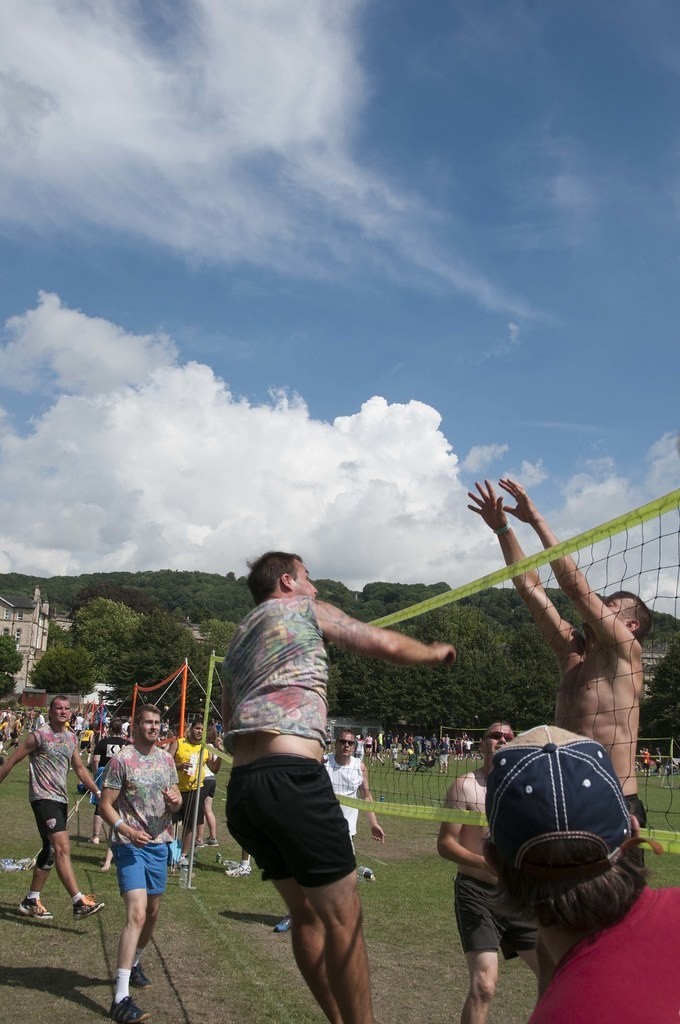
[{"xmin": 409, "ymin": 754, "xmax": 417, "ymax": 766}]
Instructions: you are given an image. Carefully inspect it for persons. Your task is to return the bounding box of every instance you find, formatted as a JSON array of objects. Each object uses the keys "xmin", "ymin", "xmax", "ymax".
[
  {"xmin": 324, "ymin": 730, "xmax": 483, "ymax": 773},
  {"xmin": 222, "ymin": 551, "xmax": 457, "ymax": 1024},
  {"xmin": 0, "ymin": 695, "xmax": 105, "ymax": 922},
  {"xmin": 437, "ymin": 719, "xmax": 538, "ymax": 1024},
  {"xmin": 98, "ymin": 704, "xmax": 182, "ymax": 1024},
  {"xmin": 467, "ymin": 477, "xmax": 647, "ymax": 869},
  {"xmin": 90, "ymin": 708, "xmax": 253, "ymax": 878},
  {"xmin": 635, "ymin": 747, "xmax": 680, "ymax": 777},
  {"xmin": 0, "ymin": 706, "xmax": 95, "ymax": 766},
  {"xmin": 273, "ymin": 729, "xmax": 386, "ymax": 933},
  {"xmin": 483, "ymin": 724, "xmax": 680, "ymax": 1024}
]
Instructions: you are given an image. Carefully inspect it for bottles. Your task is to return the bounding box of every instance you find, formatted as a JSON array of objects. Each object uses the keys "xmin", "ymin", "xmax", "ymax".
[
  {"xmin": 179, "ymin": 857, "xmax": 189, "ymax": 889},
  {"xmin": 223, "ymin": 860, "xmax": 240, "ymax": 867},
  {"xmin": 357, "ymin": 865, "xmax": 376, "ymax": 882},
  {"xmin": 1, "ymin": 858, "xmax": 31, "ymax": 870},
  {"xmin": 273, "ymin": 910, "xmax": 292, "ymax": 933},
  {"xmin": 188, "ymin": 754, "xmax": 197, "ymax": 777}
]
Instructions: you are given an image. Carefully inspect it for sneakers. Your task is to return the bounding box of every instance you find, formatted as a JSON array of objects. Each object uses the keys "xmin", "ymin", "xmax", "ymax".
[
  {"xmin": 130, "ymin": 964, "xmax": 153, "ymax": 988},
  {"xmin": 73, "ymin": 894, "xmax": 105, "ymax": 922},
  {"xmin": 225, "ymin": 863, "xmax": 252, "ymax": 878},
  {"xmin": 109, "ymin": 996, "xmax": 153, "ymax": 1024},
  {"xmin": 273, "ymin": 914, "xmax": 293, "ymax": 932},
  {"xmin": 18, "ymin": 895, "xmax": 54, "ymax": 919},
  {"xmin": 195, "ymin": 836, "xmax": 219, "ymax": 847}
]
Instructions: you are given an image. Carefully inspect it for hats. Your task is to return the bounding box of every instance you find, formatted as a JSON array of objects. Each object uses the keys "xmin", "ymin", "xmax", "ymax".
[{"xmin": 484, "ymin": 725, "xmax": 664, "ymax": 887}]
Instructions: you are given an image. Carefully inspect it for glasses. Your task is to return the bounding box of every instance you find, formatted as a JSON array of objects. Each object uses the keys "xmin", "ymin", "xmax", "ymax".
[
  {"xmin": 482, "ymin": 731, "xmax": 514, "ymax": 742},
  {"xmin": 336, "ymin": 739, "xmax": 355, "ymax": 746}
]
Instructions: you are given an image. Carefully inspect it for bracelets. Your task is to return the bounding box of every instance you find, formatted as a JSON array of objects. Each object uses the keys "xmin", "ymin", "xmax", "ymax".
[
  {"xmin": 113, "ymin": 819, "xmax": 125, "ymax": 830},
  {"xmin": 493, "ymin": 522, "xmax": 511, "ymax": 535},
  {"xmin": 93, "ymin": 791, "xmax": 101, "ymax": 796}
]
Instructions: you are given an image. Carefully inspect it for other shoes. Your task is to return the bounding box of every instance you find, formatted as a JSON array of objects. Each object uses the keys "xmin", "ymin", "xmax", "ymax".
[
  {"xmin": 448, "ymin": 754, "xmax": 467, "ymax": 761},
  {"xmin": 439, "ymin": 770, "xmax": 447, "ymax": 773},
  {"xmin": 88, "ymin": 835, "xmax": 99, "ymax": 844},
  {"xmin": 3, "ymin": 751, "xmax": 8, "ymax": 755}
]
